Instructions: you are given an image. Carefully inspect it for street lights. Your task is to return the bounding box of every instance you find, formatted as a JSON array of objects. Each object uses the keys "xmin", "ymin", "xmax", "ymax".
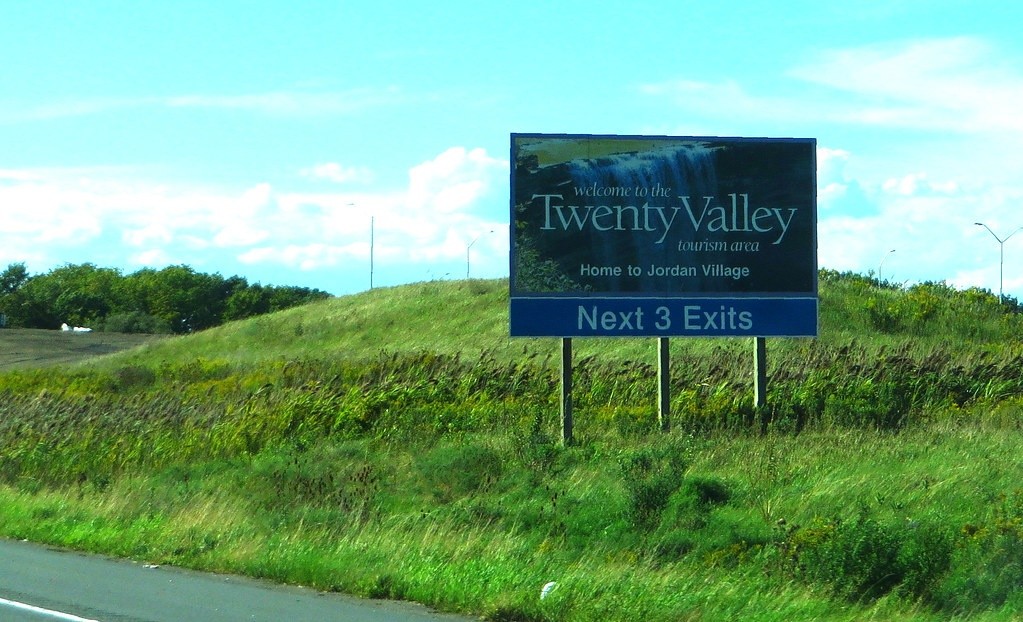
[
  {"xmin": 975, "ymin": 222, "xmax": 1023, "ymax": 305},
  {"xmin": 877, "ymin": 250, "xmax": 895, "ymax": 289}
]
